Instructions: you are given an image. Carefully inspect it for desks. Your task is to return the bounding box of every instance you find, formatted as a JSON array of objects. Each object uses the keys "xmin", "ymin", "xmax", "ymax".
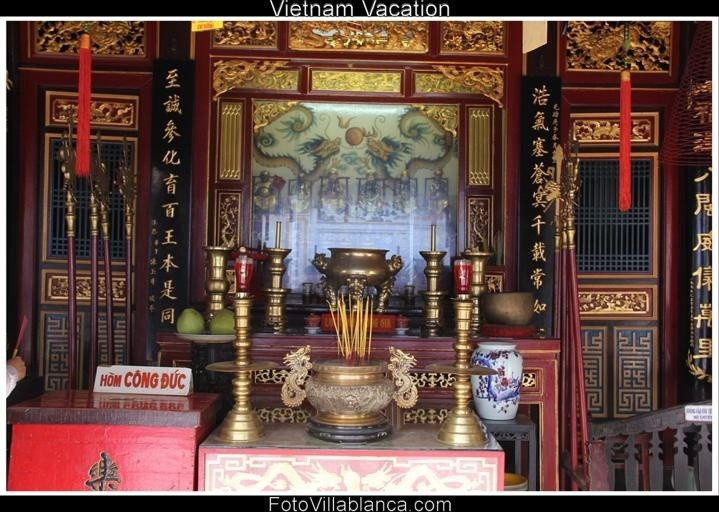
[
  {"xmin": 198, "ymin": 422, "xmax": 506, "ymax": 492},
  {"xmin": 155, "ymin": 314, "xmax": 562, "ymax": 491},
  {"xmin": 6, "ymin": 390, "xmax": 223, "ymax": 492},
  {"xmin": 481, "ymin": 414, "xmax": 537, "ymax": 491}
]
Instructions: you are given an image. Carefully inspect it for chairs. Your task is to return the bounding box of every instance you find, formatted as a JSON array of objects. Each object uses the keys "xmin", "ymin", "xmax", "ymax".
[{"xmin": 253, "ymin": 176, "xmax": 456, "ymax": 272}]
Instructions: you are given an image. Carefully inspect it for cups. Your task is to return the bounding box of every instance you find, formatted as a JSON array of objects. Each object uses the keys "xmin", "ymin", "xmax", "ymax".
[
  {"xmin": 304, "ymin": 313, "xmax": 321, "ymax": 327},
  {"xmin": 394, "ymin": 313, "xmax": 410, "ymax": 329}
]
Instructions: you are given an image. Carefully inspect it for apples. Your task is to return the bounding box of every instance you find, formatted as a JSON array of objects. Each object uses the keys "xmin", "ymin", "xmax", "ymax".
[
  {"xmin": 209, "ymin": 308, "xmax": 237, "ymax": 334},
  {"xmin": 176, "ymin": 308, "xmax": 204, "ymax": 335}
]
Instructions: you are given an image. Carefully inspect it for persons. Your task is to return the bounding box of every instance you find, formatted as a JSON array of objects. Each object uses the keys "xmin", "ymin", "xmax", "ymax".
[{"xmin": 6, "ymin": 356, "xmax": 27, "ymax": 399}]
